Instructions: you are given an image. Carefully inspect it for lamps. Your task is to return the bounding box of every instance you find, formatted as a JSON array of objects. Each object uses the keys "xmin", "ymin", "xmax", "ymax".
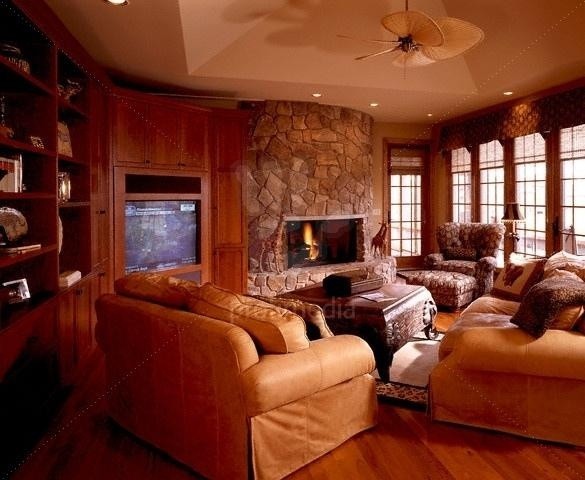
[{"xmin": 500, "ymin": 201, "xmax": 526, "ymax": 261}]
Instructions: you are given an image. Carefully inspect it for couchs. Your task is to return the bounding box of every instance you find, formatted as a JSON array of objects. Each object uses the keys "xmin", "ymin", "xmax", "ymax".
[
  {"xmin": 424, "ymin": 253, "xmax": 585, "ymax": 451},
  {"xmin": 92, "ymin": 270, "xmax": 379, "ymax": 480}
]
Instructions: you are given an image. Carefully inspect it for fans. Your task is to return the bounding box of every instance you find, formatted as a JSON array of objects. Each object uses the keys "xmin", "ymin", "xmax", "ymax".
[{"xmin": 334, "ymin": 0, "xmax": 484, "ymax": 71}]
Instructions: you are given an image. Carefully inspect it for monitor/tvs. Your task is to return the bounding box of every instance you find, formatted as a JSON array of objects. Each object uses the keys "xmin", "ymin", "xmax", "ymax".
[{"xmin": 124, "ymin": 200, "xmax": 201, "ymax": 275}]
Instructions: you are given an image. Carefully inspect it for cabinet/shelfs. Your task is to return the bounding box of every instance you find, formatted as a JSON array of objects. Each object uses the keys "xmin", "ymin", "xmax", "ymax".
[{"xmin": 114, "ymin": 93, "xmax": 210, "ymax": 170}]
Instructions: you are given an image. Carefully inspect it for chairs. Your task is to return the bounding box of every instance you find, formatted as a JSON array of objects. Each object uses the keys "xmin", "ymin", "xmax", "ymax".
[{"xmin": 405, "ymin": 221, "xmax": 506, "ymax": 297}]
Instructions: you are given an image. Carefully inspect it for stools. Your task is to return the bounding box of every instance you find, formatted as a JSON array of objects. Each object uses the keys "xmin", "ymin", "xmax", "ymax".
[{"xmin": 404, "ymin": 270, "xmax": 479, "ymax": 315}]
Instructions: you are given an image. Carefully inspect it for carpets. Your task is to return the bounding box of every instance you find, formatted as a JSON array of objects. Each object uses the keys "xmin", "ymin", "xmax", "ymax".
[{"xmin": 375, "ymin": 326, "xmax": 447, "ymax": 416}]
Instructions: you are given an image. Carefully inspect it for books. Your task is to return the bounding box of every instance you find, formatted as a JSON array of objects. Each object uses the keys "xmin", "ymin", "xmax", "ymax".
[
  {"xmin": 0, "ymin": 157, "xmax": 19, "ymax": 195},
  {"xmin": 59, "ymin": 269, "xmax": 81, "ymax": 288},
  {"xmin": 360, "ymin": 290, "xmax": 397, "ymax": 306},
  {"xmin": 0, "ymin": 153, "xmax": 23, "ymax": 194},
  {"xmin": 4, "ymin": 242, "xmax": 41, "ymax": 254}
]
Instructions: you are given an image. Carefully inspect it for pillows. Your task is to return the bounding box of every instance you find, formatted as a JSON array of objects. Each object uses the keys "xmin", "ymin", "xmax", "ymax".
[
  {"xmin": 489, "ymin": 257, "xmax": 548, "ymax": 302},
  {"xmin": 243, "ymin": 291, "xmax": 335, "ymax": 341}
]
